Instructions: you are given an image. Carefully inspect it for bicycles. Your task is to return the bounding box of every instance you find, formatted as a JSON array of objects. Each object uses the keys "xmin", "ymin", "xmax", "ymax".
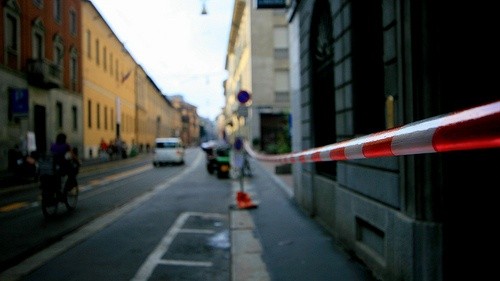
[{"xmin": 37, "ymin": 156, "xmax": 79, "ymax": 218}]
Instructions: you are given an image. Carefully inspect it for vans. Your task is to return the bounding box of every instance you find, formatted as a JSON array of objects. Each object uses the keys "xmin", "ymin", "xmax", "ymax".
[{"xmin": 153, "ymin": 138, "xmax": 186, "ymax": 166}]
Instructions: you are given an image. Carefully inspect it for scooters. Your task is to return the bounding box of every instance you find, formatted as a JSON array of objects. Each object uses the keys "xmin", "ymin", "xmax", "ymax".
[{"xmin": 201, "ymin": 140, "xmax": 230, "ymax": 179}]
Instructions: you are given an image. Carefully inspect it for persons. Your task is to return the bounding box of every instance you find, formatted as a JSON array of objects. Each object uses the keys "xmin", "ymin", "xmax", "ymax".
[
  {"xmin": 94, "ymin": 134, "xmax": 142, "ymax": 161},
  {"xmin": 46, "ymin": 131, "xmax": 80, "ymax": 202},
  {"xmin": 7, "ymin": 138, "xmax": 42, "ymax": 183}
]
